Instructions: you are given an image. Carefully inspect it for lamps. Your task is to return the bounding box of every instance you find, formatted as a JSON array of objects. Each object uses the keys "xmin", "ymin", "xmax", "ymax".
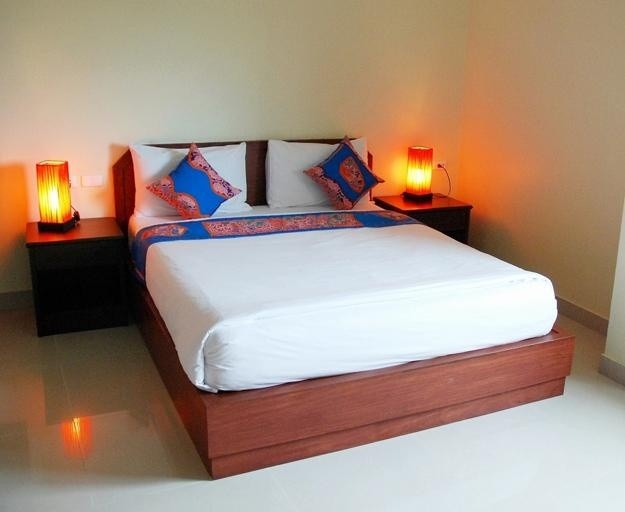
[
  {"xmin": 29, "ymin": 158, "xmax": 80, "ymax": 233},
  {"xmin": 400, "ymin": 144, "xmax": 435, "ymax": 205}
]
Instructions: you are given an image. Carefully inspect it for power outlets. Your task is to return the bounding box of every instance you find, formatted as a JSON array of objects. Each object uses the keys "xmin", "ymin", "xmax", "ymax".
[{"xmin": 433, "ymin": 160, "xmax": 447, "ymax": 172}]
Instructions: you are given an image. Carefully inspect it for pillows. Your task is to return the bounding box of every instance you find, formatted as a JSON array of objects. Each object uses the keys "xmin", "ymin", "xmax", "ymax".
[
  {"xmin": 127, "ymin": 141, "xmax": 253, "ymax": 217},
  {"xmin": 146, "ymin": 144, "xmax": 244, "ymax": 219},
  {"xmin": 264, "ymin": 138, "xmax": 376, "ymax": 211},
  {"xmin": 302, "ymin": 137, "xmax": 387, "ymax": 213}
]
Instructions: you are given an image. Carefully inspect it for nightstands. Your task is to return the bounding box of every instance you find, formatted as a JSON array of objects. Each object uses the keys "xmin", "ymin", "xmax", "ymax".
[
  {"xmin": 375, "ymin": 190, "xmax": 475, "ymax": 246},
  {"xmin": 25, "ymin": 215, "xmax": 135, "ymax": 338}
]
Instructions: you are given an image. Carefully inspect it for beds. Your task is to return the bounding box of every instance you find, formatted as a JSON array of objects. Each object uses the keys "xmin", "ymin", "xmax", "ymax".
[{"xmin": 109, "ymin": 137, "xmax": 578, "ymax": 483}]
